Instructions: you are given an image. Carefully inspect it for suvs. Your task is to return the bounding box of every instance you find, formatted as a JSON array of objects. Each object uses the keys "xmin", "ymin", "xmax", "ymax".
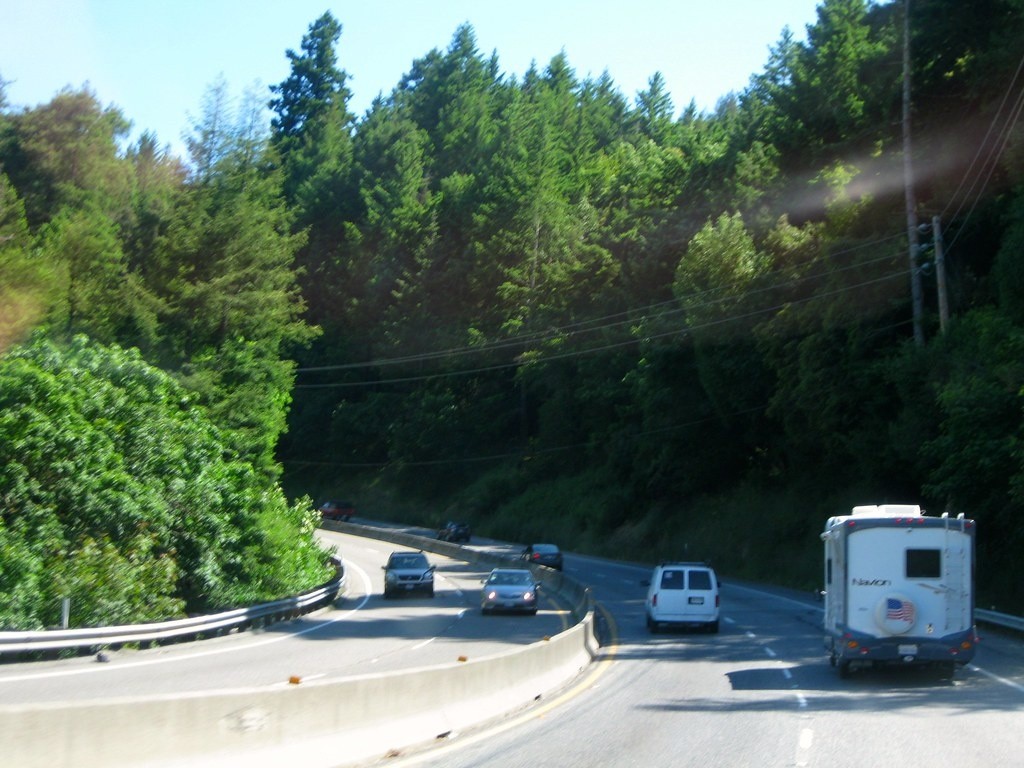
[{"xmin": 381, "ymin": 550, "xmax": 436, "ymax": 597}]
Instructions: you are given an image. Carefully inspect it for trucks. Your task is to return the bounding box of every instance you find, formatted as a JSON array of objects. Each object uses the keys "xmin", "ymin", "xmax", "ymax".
[{"xmin": 821, "ymin": 504, "xmax": 978, "ymax": 677}]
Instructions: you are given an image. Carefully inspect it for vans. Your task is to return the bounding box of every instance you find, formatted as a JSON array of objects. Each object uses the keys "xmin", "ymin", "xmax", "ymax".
[{"xmin": 638, "ymin": 559, "xmax": 722, "ymax": 634}]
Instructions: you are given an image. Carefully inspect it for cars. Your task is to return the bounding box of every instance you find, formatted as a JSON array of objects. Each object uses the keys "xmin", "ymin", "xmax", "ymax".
[
  {"xmin": 316, "ymin": 500, "xmax": 357, "ymax": 521},
  {"xmin": 520, "ymin": 543, "xmax": 563, "ymax": 571},
  {"xmin": 481, "ymin": 568, "xmax": 542, "ymax": 616},
  {"xmin": 436, "ymin": 520, "xmax": 472, "ymax": 542}
]
